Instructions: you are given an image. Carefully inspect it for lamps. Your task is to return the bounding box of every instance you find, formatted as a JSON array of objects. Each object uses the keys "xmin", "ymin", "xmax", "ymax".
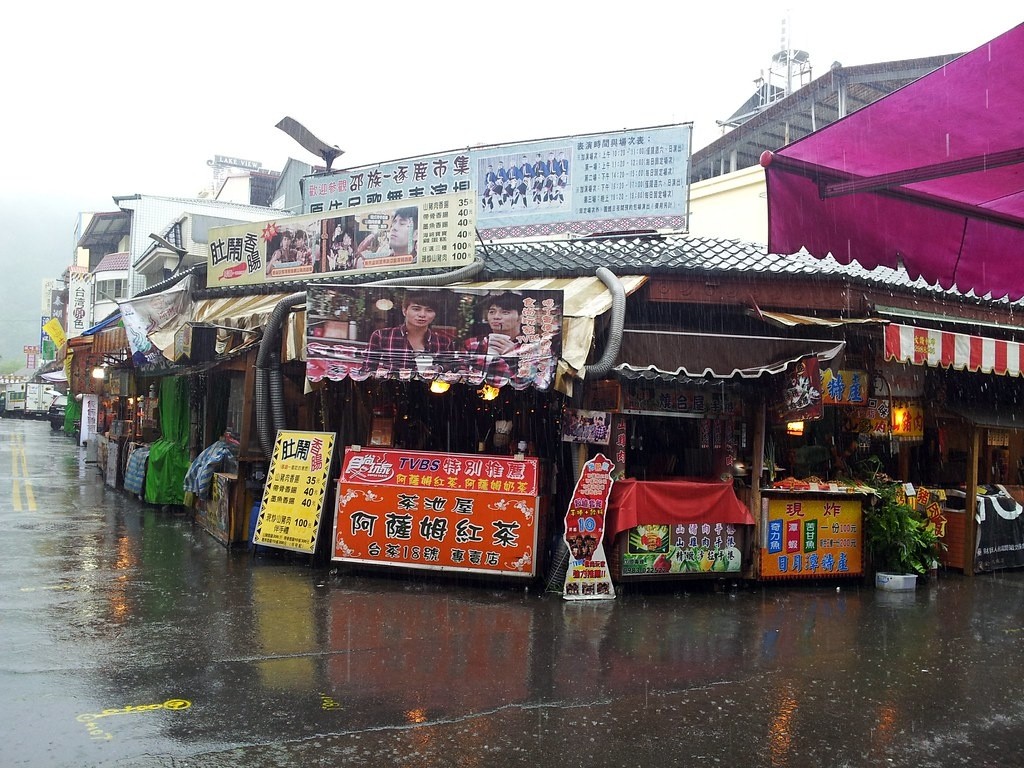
[
  {"xmin": 93, "ymin": 354, "xmax": 105, "ymax": 379},
  {"xmin": 786, "ymin": 422, "xmax": 803, "ymax": 436},
  {"xmin": 477, "ymin": 382, "xmax": 500, "ymax": 400}
]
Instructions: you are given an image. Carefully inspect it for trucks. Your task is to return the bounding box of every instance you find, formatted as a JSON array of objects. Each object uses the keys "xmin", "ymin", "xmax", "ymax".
[{"xmin": 0, "ymin": 382, "xmax": 54, "ymax": 420}]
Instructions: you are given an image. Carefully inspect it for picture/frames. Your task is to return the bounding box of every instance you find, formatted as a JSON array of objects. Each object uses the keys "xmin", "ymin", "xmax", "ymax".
[{"xmin": 367, "ymin": 415, "xmax": 395, "ymax": 448}]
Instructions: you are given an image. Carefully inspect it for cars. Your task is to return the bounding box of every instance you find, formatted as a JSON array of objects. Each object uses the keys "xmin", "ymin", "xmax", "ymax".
[{"xmin": 47, "ymin": 394, "xmax": 68, "ymax": 430}]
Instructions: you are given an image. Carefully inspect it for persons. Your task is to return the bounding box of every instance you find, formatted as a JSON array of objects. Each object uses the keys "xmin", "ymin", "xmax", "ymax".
[
  {"xmin": 327, "ymin": 231, "xmax": 354, "ymax": 270},
  {"xmin": 266, "ymin": 230, "xmax": 320, "ymax": 274},
  {"xmin": 389, "ymin": 206, "xmax": 417, "ymax": 263},
  {"xmin": 564, "ymin": 414, "xmax": 607, "ymax": 441},
  {"xmin": 482, "ymin": 152, "xmax": 568, "ymax": 213},
  {"xmin": 571, "ymin": 535, "xmax": 596, "ymax": 559},
  {"xmin": 362, "ymin": 290, "xmax": 455, "ymax": 378},
  {"xmin": 446, "ymin": 292, "xmax": 557, "ymax": 388}
]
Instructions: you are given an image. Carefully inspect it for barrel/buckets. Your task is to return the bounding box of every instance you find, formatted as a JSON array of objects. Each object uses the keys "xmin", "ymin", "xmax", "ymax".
[{"xmin": 248, "ymin": 502, "xmax": 277, "ymax": 554}]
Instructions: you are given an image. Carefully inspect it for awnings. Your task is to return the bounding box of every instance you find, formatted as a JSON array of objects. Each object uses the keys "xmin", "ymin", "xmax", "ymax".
[
  {"xmin": 760, "ymin": 22, "xmax": 1024, "ymax": 302},
  {"xmin": 604, "ymin": 331, "xmax": 845, "ymax": 380},
  {"xmin": 55, "ymin": 322, "xmax": 133, "ymax": 366},
  {"xmin": 747, "ymin": 307, "xmax": 1024, "ymax": 377},
  {"xmin": 149, "ymin": 292, "xmax": 305, "ymax": 363},
  {"xmin": 306, "ymin": 274, "xmax": 643, "ymax": 395}
]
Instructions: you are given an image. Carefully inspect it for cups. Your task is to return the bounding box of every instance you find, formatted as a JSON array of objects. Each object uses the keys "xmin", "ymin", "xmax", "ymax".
[
  {"xmin": 487, "ymin": 333, "xmax": 510, "ymax": 354},
  {"xmin": 415, "ymin": 356, "xmax": 433, "ymax": 377}
]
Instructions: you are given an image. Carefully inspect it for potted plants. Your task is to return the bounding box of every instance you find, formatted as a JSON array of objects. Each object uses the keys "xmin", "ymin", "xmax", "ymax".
[{"xmin": 861, "ymin": 482, "xmax": 949, "ymax": 593}]
